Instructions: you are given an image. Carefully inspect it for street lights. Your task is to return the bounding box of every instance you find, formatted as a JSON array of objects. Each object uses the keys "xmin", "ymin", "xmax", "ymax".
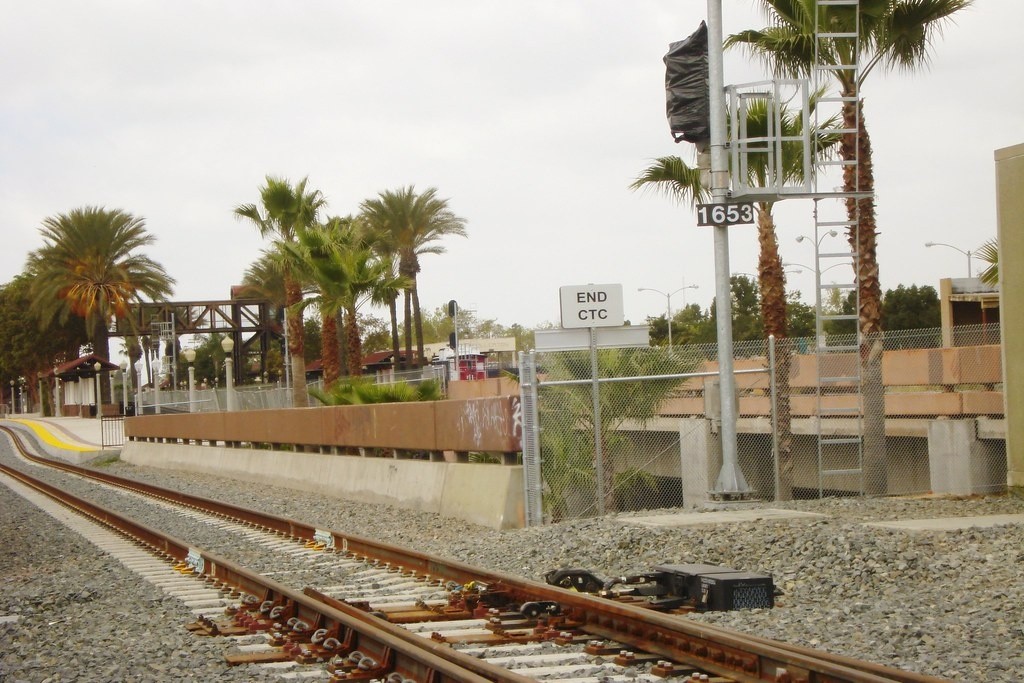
[
  {"xmin": 119, "ymin": 360, "xmax": 129, "ymax": 415},
  {"xmin": 134, "ymin": 360, "xmax": 144, "ymax": 416},
  {"xmin": 185, "ymin": 348, "xmax": 197, "ymax": 414},
  {"xmin": 10, "ymin": 380, "xmax": 15, "ymax": 414},
  {"xmin": 637, "ymin": 285, "xmax": 699, "ymax": 352},
  {"xmin": 925, "ymin": 242, "xmax": 971, "ymax": 279},
  {"xmin": 151, "ymin": 358, "xmax": 161, "ymax": 414},
  {"xmin": 796, "ymin": 229, "xmax": 838, "ymax": 337},
  {"xmin": 222, "ymin": 336, "xmax": 235, "ymax": 411},
  {"xmin": 37, "ymin": 371, "xmax": 45, "ymax": 418},
  {"xmin": 94, "ymin": 362, "xmax": 105, "ymax": 420},
  {"xmin": 54, "ymin": 367, "xmax": 62, "ymax": 417}
]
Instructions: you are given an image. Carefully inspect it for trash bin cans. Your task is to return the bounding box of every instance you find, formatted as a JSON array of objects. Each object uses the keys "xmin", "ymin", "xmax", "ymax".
[{"xmin": 89, "ymin": 403, "xmax": 96, "ymax": 416}]
